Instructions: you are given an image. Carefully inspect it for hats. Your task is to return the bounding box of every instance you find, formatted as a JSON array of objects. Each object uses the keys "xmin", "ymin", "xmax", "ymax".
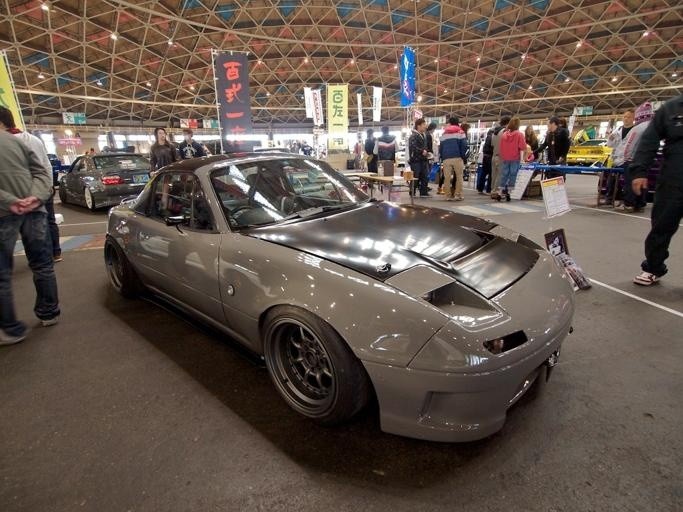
[{"xmin": 634, "ymin": 102, "xmax": 655, "ymax": 120}]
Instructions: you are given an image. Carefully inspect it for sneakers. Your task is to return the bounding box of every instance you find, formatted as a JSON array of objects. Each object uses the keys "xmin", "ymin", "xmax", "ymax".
[
  {"xmin": 633, "ymin": 272, "xmax": 665, "ymax": 286},
  {"xmin": 476, "ymin": 188, "xmax": 515, "ymax": 202},
  {"xmin": 408, "ymin": 187, "xmax": 466, "ymax": 202},
  {"xmin": 599, "ymin": 198, "xmax": 645, "ymax": 214},
  {"xmin": 0, "ymin": 253, "xmax": 64, "ymax": 346}
]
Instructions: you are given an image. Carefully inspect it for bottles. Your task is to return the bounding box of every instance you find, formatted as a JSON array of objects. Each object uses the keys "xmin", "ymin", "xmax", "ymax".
[
  {"xmin": 377, "ymin": 162, "xmax": 384, "ymax": 177},
  {"xmin": 399, "ymin": 165, "xmax": 404, "ymax": 177}
]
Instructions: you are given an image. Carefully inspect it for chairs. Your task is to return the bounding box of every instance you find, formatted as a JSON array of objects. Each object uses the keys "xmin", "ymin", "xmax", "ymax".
[{"xmin": 245, "ymin": 172, "xmax": 300, "ymax": 219}]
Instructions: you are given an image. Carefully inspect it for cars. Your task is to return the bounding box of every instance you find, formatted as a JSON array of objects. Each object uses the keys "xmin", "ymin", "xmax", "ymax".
[
  {"xmin": 567, "ymin": 141, "xmax": 616, "ymax": 168},
  {"xmin": 48, "ymin": 155, "xmax": 72, "ymax": 178},
  {"xmin": 598, "ymin": 146, "xmax": 665, "ymax": 199},
  {"xmin": 60, "ymin": 152, "xmax": 157, "ymax": 212}
]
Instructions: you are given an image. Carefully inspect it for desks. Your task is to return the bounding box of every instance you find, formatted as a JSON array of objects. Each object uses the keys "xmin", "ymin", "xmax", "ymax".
[
  {"xmin": 352, "ymin": 173, "xmax": 418, "ymax": 204},
  {"xmin": 475, "ymin": 162, "xmax": 626, "ymax": 207}
]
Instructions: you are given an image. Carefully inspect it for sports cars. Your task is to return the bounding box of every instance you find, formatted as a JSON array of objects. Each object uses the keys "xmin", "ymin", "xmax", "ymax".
[{"xmin": 105, "ymin": 152, "xmax": 576, "ymax": 443}]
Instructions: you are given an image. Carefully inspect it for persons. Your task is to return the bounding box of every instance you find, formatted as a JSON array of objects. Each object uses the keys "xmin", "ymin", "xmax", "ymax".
[
  {"xmin": 84, "ymin": 151, "xmax": 89, "ymax": 155},
  {"xmin": 0, "ymin": 127, "xmax": 61, "ymax": 348},
  {"xmin": 176, "ymin": 128, "xmax": 208, "ymax": 158},
  {"xmin": 89, "ymin": 147, "xmax": 95, "ymax": 155},
  {"xmin": 598, "ymin": 109, "xmax": 637, "ymax": 205},
  {"xmin": 267, "ymin": 139, "xmax": 312, "ymax": 156},
  {"xmin": 353, "ymin": 127, "xmax": 397, "ymax": 185},
  {"xmin": 152, "ymin": 126, "xmax": 180, "ymax": 169},
  {"xmin": 621, "ymin": 102, "xmax": 661, "ymax": 211},
  {"xmin": 477, "ymin": 115, "xmax": 571, "ymax": 202},
  {"xmin": 631, "ymin": 93, "xmax": 682, "ymax": 285},
  {"xmin": 409, "ymin": 117, "xmax": 474, "ymax": 201},
  {"xmin": 0, "ymin": 104, "xmax": 61, "ymax": 262}
]
